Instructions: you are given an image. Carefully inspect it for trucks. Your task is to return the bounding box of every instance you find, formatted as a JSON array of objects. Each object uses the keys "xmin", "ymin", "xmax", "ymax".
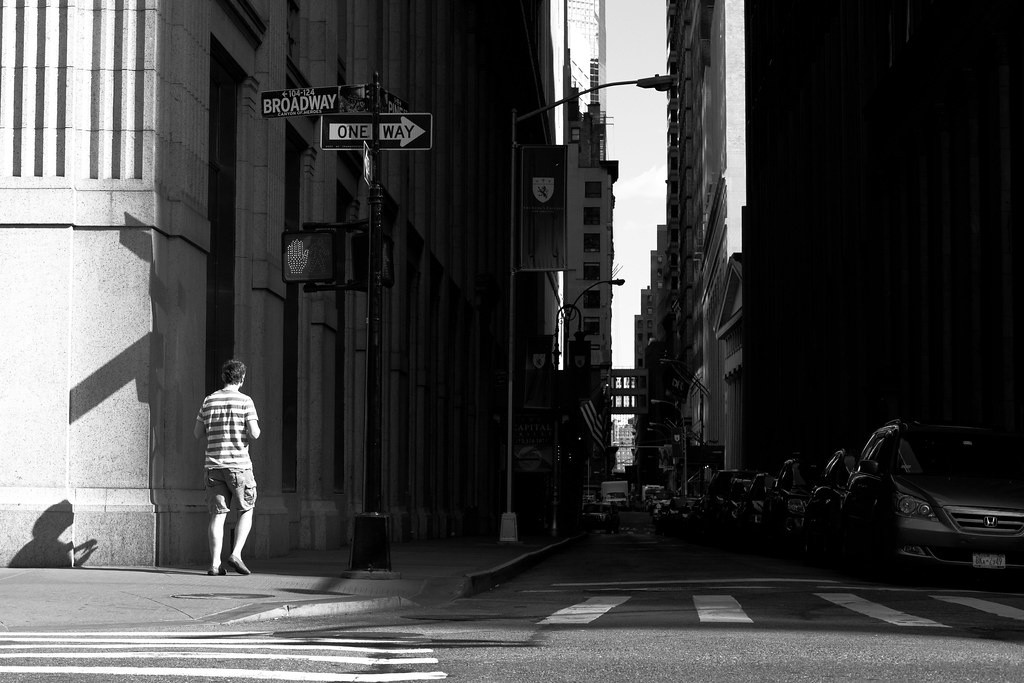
[{"xmin": 600, "ymin": 481, "xmax": 629, "ymax": 510}]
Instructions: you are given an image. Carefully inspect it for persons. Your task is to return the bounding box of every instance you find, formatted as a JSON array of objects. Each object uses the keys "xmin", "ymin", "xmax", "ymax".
[{"xmin": 194, "ymin": 360, "xmax": 262, "ymax": 576}]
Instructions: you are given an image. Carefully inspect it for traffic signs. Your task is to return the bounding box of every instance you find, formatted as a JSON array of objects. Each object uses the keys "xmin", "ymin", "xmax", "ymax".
[
  {"xmin": 320, "ymin": 113, "xmax": 433, "ymax": 150},
  {"xmin": 260, "ymin": 83, "xmax": 373, "ymax": 118},
  {"xmin": 378, "ymin": 86, "xmax": 410, "ymax": 113},
  {"xmin": 362, "ymin": 140, "xmax": 372, "ymax": 186}
]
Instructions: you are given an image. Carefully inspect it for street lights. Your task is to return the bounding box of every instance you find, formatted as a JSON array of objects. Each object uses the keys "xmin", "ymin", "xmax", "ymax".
[{"xmin": 499, "ymin": 73, "xmax": 709, "ymax": 542}]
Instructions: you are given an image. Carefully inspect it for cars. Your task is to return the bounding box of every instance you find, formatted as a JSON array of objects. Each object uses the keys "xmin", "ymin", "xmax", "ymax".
[{"xmin": 645, "ymin": 414, "xmax": 1024, "ymax": 589}]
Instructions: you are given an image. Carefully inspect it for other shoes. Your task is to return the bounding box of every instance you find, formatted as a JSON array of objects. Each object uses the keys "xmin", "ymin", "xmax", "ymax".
[
  {"xmin": 227, "ymin": 555, "xmax": 250, "ymax": 575},
  {"xmin": 207, "ymin": 566, "xmax": 226, "ymax": 576}
]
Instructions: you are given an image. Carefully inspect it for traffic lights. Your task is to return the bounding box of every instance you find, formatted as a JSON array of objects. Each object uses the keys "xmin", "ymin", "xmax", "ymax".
[
  {"xmin": 282, "ymin": 229, "xmax": 335, "ymax": 285},
  {"xmin": 378, "ymin": 236, "xmax": 395, "ymax": 290}
]
